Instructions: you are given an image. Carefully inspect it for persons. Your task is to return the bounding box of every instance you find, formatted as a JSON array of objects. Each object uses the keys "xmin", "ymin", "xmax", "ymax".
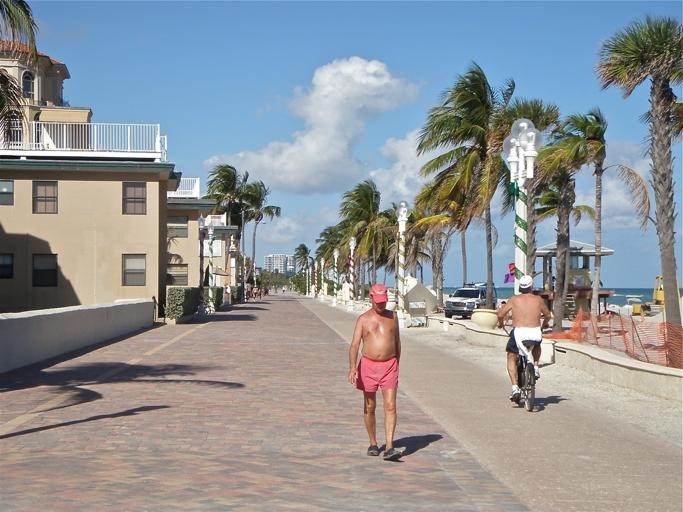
[
  {"xmin": 251, "ymin": 286, "xmax": 269, "ymax": 303},
  {"xmin": 497, "ymin": 275, "xmax": 551, "ymax": 403},
  {"xmin": 496, "ymin": 301, "xmax": 506, "ymax": 310},
  {"xmin": 347, "ymin": 283, "xmax": 402, "ymax": 461},
  {"xmin": 544, "ymin": 273, "xmax": 557, "ymax": 291}
]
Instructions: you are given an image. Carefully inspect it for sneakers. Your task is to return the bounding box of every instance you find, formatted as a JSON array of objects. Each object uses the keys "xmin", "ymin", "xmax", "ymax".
[
  {"xmin": 534, "ymin": 369, "xmax": 540, "ymax": 378},
  {"xmin": 383, "ymin": 448, "xmax": 402, "ymax": 460},
  {"xmin": 509, "ymin": 387, "xmax": 520, "ymax": 400},
  {"xmin": 367, "ymin": 446, "xmax": 378, "ymax": 456}
]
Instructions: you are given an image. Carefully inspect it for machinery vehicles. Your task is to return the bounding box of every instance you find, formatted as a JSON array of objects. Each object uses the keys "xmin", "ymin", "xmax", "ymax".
[{"xmin": 631, "ymin": 275, "xmax": 665, "ymax": 317}]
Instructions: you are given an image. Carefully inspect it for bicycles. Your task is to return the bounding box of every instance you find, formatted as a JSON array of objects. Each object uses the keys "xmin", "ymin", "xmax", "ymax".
[{"xmin": 496, "ymin": 324, "xmax": 556, "ymax": 412}]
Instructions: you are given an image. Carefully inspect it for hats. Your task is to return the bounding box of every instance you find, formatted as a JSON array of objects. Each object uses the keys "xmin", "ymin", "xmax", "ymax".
[
  {"xmin": 519, "ymin": 275, "xmax": 533, "ymax": 288},
  {"xmin": 369, "ymin": 284, "xmax": 388, "ymax": 303}
]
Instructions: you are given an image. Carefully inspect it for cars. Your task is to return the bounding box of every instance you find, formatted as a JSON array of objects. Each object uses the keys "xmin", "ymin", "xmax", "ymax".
[{"xmin": 444, "ymin": 281, "xmax": 497, "ymax": 319}]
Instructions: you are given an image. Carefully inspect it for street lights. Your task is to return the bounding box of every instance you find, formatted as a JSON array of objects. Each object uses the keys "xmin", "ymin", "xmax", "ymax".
[
  {"xmin": 205, "ymin": 219, "xmax": 215, "ymax": 312},
  {"xmin": 244, "ymin": 255, "xmax": 256, "ymax": 303},
  {"xmin": 395, "ymin": 200, "xmax": 412, "ymax": 320},
  {"xmin": 302, "ymin": 248, "xmax": 340, "ymax": 297},
  {"xmin": 500, "ymin": 118, "xmax": 543, "ymax": 296},
  {"xmin": 349, "ymin": 237, "xmax": 357, "ymax": 299}
]
